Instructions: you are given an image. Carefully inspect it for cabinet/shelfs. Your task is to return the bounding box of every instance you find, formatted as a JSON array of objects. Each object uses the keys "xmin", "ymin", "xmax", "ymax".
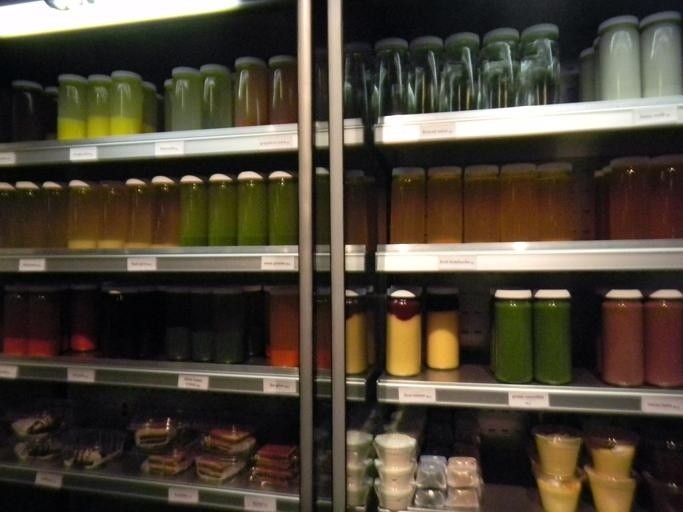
[
  {"xmin": 0, "ymin": 0, "xmax": 334, "ymax": 509},
  {"xmin": 319, "ymin": 0, "xmax": 682, "ymax": 512}
]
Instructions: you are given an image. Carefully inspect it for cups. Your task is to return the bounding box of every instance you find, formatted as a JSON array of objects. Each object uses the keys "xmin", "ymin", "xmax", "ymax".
[{"xmin": 345, "ymin": 410, "xmax": 682, "ymax": 510}]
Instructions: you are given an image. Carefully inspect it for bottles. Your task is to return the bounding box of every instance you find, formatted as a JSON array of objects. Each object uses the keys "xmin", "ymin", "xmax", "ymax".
[
  {"xmin": 339, "ymin": 272, "xmax": 683, "ymax": 394},
  {"xmin": 343, "ymin": 139, "xmax": 683, "ymax": 245},
  {"xmin": 3, "ymin": 159, "xmax": 344, "ymax": 262},
  {"xmin": 4, "ymin": 281, "xmax": 333, "ymax": 380},
  {"xmin": 346, "ymin": 10, "xmax": 683, "ymax": 115},
  {"xmin": 0, "ymin": 47, "xmax": 328, "ymax": 142}
]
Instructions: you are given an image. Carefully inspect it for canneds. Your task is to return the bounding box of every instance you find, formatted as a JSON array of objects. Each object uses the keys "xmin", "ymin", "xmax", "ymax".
[{"xmin": 1, "ymin": 11, "xmax": 682, "ymax": 389}]
